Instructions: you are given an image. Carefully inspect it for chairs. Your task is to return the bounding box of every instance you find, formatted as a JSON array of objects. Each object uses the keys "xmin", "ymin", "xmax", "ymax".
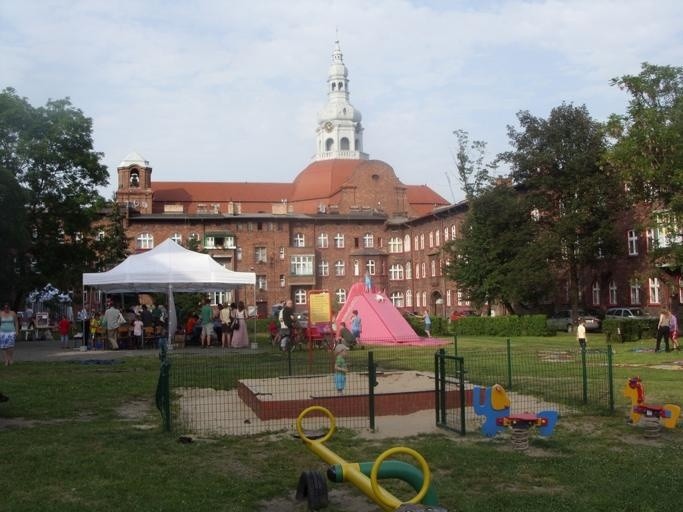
[
  {"xmin": 183, "ymin": 327, "xmax": 193, "ymax": 346},
  {"xmin": 93, "ymin": 327, "xmax": 108, "ymax": 351},
  {"xmin": 115, "ymin": 325, "xmax": 167, "ymax": 349}
]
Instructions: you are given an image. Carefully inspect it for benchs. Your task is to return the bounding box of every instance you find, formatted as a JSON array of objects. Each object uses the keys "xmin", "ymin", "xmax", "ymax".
[{"xmin": 72, "ymin": 332, "xmax": 84, "ymax": 348}]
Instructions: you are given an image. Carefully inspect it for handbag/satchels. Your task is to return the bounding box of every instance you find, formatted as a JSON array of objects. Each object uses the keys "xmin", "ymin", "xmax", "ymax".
[{"xmin": 230, "ymin": 318, "xmax": 240, "ymax": 331}]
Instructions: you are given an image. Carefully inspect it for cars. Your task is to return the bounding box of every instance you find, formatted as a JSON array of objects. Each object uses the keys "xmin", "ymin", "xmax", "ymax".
[
  {"xmin": 448, "ymin": 310, "xmax": 481, "ymax": 322},
  {"xmin": 606, "ymin": 307, "xmax": 655, "ymax": 320},
  {"xmin": 547, "ymin": 309, "xmax": 601, "ymax": 332}
]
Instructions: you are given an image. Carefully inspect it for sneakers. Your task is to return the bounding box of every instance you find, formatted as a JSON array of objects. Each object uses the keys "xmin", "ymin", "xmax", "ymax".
[
  {"xmin": 655, "ymin": 344, "xmax": 681, "ymax": 354},
  {"xmin": 200, "ymin": 343, "xmax": 214, "ymax": 350}
]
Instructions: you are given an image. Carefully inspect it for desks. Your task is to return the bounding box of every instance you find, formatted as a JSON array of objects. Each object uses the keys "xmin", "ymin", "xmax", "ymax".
[{"xmin": 192, "ymin": 325, "xmax": 203, "ymax": 344}]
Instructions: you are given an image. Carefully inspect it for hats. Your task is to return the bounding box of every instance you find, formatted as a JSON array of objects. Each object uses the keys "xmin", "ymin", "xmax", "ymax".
[{"xmin": 334, "ymin": 344, "xmax": 350, "ymax": 355}]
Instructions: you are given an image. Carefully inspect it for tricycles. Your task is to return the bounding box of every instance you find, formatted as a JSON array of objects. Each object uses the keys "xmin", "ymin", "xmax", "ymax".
[{"xmin": 273, "ymin": 315, "xmax": 338, "ymax": 355}]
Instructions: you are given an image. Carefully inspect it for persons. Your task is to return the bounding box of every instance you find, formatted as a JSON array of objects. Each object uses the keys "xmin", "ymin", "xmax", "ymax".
[
  {"xmin": 57, "ymin": 313, "xmax": 73, "ymax": 350},
  {"xmin": 0, "ymin": 303, "xmax": 19, "ymax": 367},
  {"xmin": 332, "ymin": 344, "xmax": 350, "ymax": 396},
  {"xmin": 339, "ymin": 321, "xmax": 364, "ymax": 350},
  {"xmin": 668, "ymin": 313, "xmax": 681, "ymax": 351},
  {"xmin": 414, "ymin": 310, "xmax": 432, "ymax": 339},
  {"xmin": 655, "ymin": 308, "xmax": 672, "ymax": 353},
  {"xmin": 278, "ymin": 299, "xmax": 301, "ymax": 353},
  {"xmin": 576, "ymin": 320, "xmax": 587, "ymax": 352},
  {"xmin": 101, "ymin": 303, "xmax": 122, "ymax": 350},
  {"xmin": 183, "ymin": 314, "xmax": 200, "ymax": 333},
  {"xmin": 89, "ymin": 314, "xmax": 100, "ymax": 351},
  {"xmin": 351, "ymin": 310, "xmax": 362, "ymax": 345},
  {"xmin": 131, "ymin": 315, "xmax": 143, "ymax": 350},
  {"xmin": 197, "ymin": 298, "xmax": 249, "ymax": 350},
  {"xmin": 140, "ymin": 304, "xmax": 154, "ymax": 337},
  {"xmin": 79, "ymin": 302, "xmax": 92, "ymax": 345}
]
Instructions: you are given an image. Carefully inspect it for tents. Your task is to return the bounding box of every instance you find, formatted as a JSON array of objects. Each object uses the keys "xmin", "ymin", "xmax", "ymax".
[{"xmin": 333, "ymin": 280, "xmax": 427, "ymax": 343}]
